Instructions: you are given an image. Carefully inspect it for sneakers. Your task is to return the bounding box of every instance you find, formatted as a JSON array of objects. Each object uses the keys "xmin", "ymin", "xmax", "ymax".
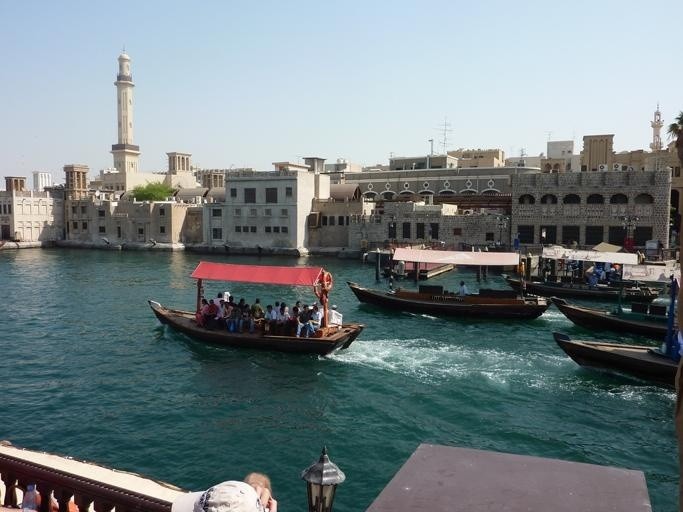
[
  {"xmin": 296, "ymin": 335, "xmax": 300, "ymax": 338},
  {"xmin": 305, "ymin": 335, "xmax": 309, "ymax": 338},
  {"xmin": 312, "ymin": 331, "xmax": 316, "ymax": 336}
]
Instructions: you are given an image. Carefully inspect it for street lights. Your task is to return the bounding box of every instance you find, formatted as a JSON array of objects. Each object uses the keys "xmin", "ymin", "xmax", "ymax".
[
  {"xmin": 389, "ymin": 221, "xmax": 395, "ymax": 244},
  {"xmin": 298, "ymin": 444, "xmax": 345, "ymax": 512},
  {"xmin": 495, "ymin": 214, "xmax": 509, "ymax": 245},
  {"xmin": 622, "ymin": 214, "xmax": 640, "ymax": 248}
]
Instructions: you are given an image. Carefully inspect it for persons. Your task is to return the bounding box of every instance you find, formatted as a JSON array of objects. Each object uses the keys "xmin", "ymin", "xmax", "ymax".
[
  {"xmin": 171, "ymin": 472, "xmax": 278, "ymax": 512},
  {"xmin": 196, "ymin": 293, "xmax": 342, "ymax": 338},
  {"xmin": 455, "ymin": 281, "xmax": 468, "ymax": 295}
]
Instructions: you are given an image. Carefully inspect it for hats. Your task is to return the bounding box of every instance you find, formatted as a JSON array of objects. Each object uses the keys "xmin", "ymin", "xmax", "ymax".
[
  {"xmin": 331, "ymin": 304, "xmax": 337, "ymax": 308},
  {"xmin": 170, "ymin": 480, "xmax": 266, "ymax": 512}
]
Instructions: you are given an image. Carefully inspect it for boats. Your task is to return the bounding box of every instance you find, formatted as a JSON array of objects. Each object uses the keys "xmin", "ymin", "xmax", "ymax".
[
  {"xmin": 548, "ymin": 294, "xmax": 680, "ymax": 342},
  {"xmin": 346, "ymin": 245, "xmax": 551, "ymax": 322},
  {"xmin": 548, "ymin": 328, "xmax": 683, "ymax": 390},
  {"xmin": 382, "ymin": 236, "xmax": 457, "ymax": 283},
  {"xmin": 144, "ymin": 259, "xmax": 365, "ymax": 359},
  {"xmin": 497, "ymin": 248, "xmax": 662, "ymax": 303}
]
